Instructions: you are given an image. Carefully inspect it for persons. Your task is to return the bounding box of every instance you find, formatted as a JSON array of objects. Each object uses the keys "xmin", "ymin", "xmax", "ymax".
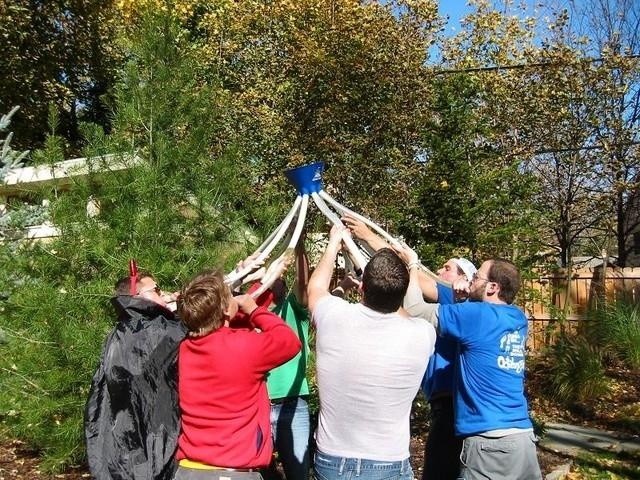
[{"xmin": 84, "ymin": 214, "xmax": 545, "ymax": 479}]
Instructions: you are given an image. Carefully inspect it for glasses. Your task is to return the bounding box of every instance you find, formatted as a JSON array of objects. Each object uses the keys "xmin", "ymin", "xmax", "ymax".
[{"xmin": 139, "ymin": 284, "xmax": 161, "ymax": 295}]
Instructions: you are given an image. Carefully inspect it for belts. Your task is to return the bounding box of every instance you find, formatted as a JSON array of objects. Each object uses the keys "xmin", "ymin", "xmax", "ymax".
[
  {"xmin": 225, "ymin": 468, "xmax": 259, "ymax": 472},
  {"xmin": 269, "ymin": 398, "xmax": 284, "ymax": 406}
]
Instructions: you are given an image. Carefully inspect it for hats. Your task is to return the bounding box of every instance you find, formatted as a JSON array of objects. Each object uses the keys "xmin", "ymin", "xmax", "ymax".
[{"xmin": 450, "ymin": 257, "xmax": 478, "ymax": 282}]
